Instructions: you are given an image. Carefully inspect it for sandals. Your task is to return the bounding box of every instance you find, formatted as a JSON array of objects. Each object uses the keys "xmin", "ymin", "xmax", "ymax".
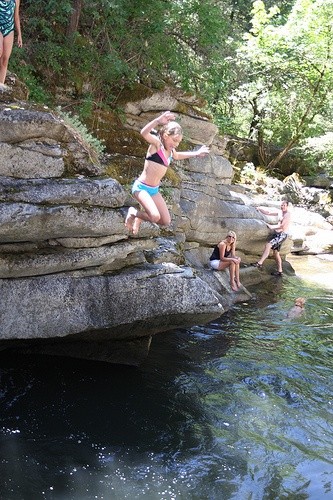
[
  {"xmin": 271, "ymin": 271, "xmax": 284, "ymax": 277},
  {"xmin": 250, "ymin": 261, "xmax": 263, "ymax": 268}
]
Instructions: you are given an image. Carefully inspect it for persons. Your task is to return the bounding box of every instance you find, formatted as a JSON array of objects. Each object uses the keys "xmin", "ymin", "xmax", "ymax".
[
  {"xmin": 250, "ymin": 200, "xmax": 289, "ymax": 276},
  {"xmin": 294, "ymin": 296, "xmax": 305, "ymax": 308},
  {"xmin": 209, "ymin": 231, "xmax": 240, "ymax": 291},
  {"xmin": 124, "ymin": 110, "xmax": 211, "ymax": 235},
  {"xmin": 0, "ymin": 0, "xmax": 23, "ymax": 85}
]
